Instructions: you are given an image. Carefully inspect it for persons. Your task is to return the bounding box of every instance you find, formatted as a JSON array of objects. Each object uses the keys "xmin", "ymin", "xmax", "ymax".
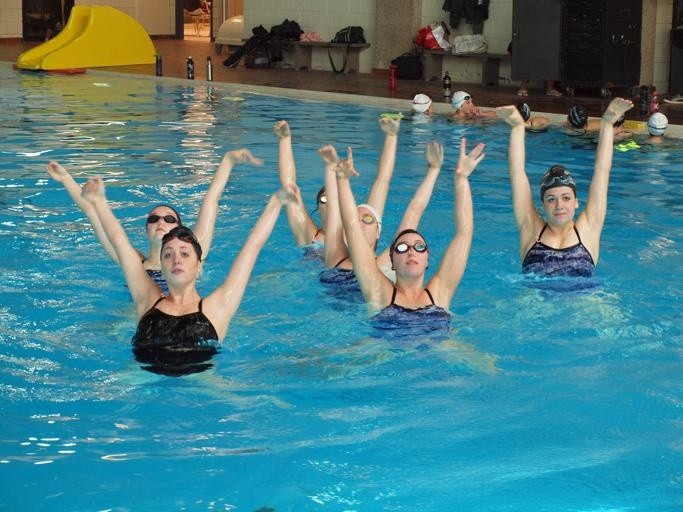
[
  {"xmin": 319, "ymin": 140, "xmax": 444, "ymax": 304},
  {"xmin": 448, "ymin": 91, "xmax": 496, "ymax": 124},
  {"xmin": 495, "ymin": 97, "xmax": 633, "ymax": 293},
  {"xmin": 516, "ymin": 103, "xmax": 549, "ymax": 134},
  {"xmin": 561, "ymin": 106, "xmax": 606, "ymax": 149},
  {"xmin": 48, "ymin": 149, "xmax": 262, "ymax": 295},
  {"xmin": 273, "ymin": 113, "xmax": 401, "ymax": 258},
  {"xmin": 613, "ymin": 116, "xmax": 639, "ymax": 151},
  {"xmin": 404, "ymin": 94, "xmax": 433, "ymax": 124},
  {"xmin": 638, "ymin": 112, "xmax": 673, "ymax": 152},
  {"xmin": 334, "ymin": 137, "xmax": 486, "ymax": 337},
  {"xmin": 81, "ymin": 173, "xmax": 301, "ymax": 377}
]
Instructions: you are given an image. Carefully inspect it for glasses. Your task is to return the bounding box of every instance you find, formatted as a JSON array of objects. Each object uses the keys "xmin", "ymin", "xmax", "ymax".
[
  {"xmin": 148, "ymin": 215, "xmax": 179, "ymax": 224},
  {"xmin": 316, "ymin": 194, "xmax": 327, "ymax": 204},
  {"xmin": 357, "ymin": 214, "xmax": 382, "ymax": 225},
  {"xmin": 463, "ymin": 95, "xmax": 473, "ymax": 100},
  {"xmin": 162, "ymin": 232, "xmax": 195, "ymax": 243},
  {"xmin": 390, "ymin": 244, "xmax": 430, "ymax": 254},
  {"xmin": 542, "ymin": 174, "xmax": 569, "ymax": 186}
]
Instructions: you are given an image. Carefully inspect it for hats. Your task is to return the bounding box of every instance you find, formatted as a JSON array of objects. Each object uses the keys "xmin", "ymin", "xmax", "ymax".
[
  {"xmin": 451, "ymin": 92, "xmax": 469, "ymax": 109},
  {"xmin": 647, "ymin": 114, "xmax": 667, "ymax": 136},
  {"xmin": 614, "ymin": 114, "xmax": 624, "ymax": 125},
  {"xmin": 162, "ymin": 228, "xmax": 202, "ymax": 257},
  {"xmin": 144, "ymin": 205, "xmax": 181, "ymax": 226},
  {"xmin": 541, "ymin": 167, "xmax": 576, "ymax": 194},
  {"xmin": 518, "ymin": 103, "xmax": 530, "ymax": 121},
  {"xmin": 358, "ymin": 204, "xmax": 381, "ymax": 232},
  {"xmin": 390, "ymin": 230, "xmax": 428, "ymax": 251},
  {"xmin": 412, "ymin": 94, "xmax": 432, "ymax": 113},
  {"xmin": 316, "ymin": 187, "xmax": 328, "ymax": 204},
  {"xmin": 568, "ymin": 105, "xmax": 588, "ymax": 129}
]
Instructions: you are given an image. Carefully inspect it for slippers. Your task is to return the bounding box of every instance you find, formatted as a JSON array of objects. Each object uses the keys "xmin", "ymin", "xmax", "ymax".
[
  {"xmin": 545, "ymin": 90, "xmax": 563, "ymax": 96},
  {"xmin": 518, "ymin": 91, "xmax": 529, "ymax": 98}
]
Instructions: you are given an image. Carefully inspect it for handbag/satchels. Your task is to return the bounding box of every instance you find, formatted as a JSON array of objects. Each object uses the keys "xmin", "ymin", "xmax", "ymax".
[
  {"xmin": 331, "ymin": 26, "xmax": 364, "ymax": 43},
  {"xmin": 452, "ymin": 35, "xmax": 487, "ymax": 54}
]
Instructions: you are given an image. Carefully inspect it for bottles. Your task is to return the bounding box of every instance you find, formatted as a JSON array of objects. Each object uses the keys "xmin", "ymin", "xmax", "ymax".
[
  {"xmin": 388, "ymin": 64, "xmax": 396, "ymax": 88},
  {"xmin": 206, "ymin": 56, "xmax": 212, "ymax": 81},
  {"xmin": 648, "ymin": 86, "xmax": 659, "ymax": 115},
  {"xmin": 156, "ymin": 52, "xmax": 161, "ymax": 76},
  {"xmin": 186, "ymin": 55, "xmax": 194, "ymax": 79},
  {"xmin": 442, "ymin": 70, "xmax": 451, "ymax": 98},
  {"xmin": 639, "ymin": 85, "xmax": 648, "ymax": 116}
]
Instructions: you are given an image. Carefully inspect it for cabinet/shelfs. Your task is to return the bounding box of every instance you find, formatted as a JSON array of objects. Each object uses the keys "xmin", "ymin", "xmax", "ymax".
[
  {"xmin": 21, "ymin": 0, "xmax": 75, "ymax": 40},
  {"xmin": 509, "ymin": 0, "xmax": 641, "ymax": 97}
]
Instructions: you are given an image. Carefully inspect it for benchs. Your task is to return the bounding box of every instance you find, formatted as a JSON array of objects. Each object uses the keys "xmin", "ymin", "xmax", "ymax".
[
  {"xmin": 415, "ymin": 47, "xmax": 510, "ymax": 87},
  {"xmin": 241, "ymin": 37, "xmax": 370, "ymax": 74}
]
